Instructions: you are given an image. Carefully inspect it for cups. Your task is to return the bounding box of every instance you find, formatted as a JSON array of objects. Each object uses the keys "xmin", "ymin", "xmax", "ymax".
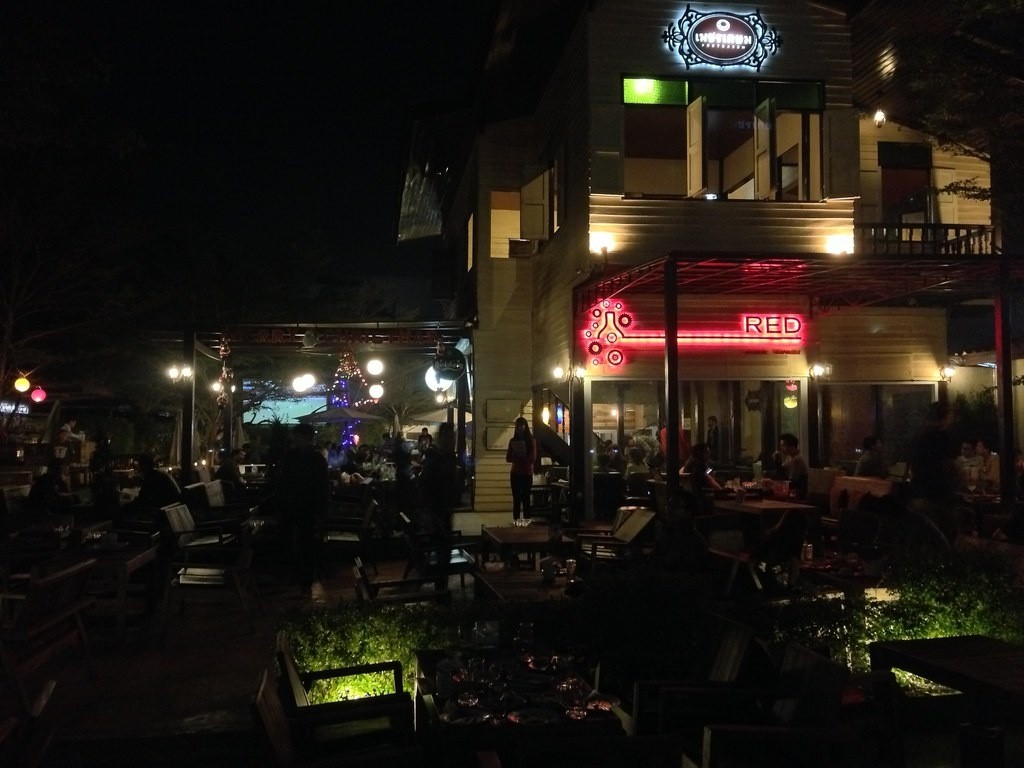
[
  {"xmin": 801, "ymin": 543, "xmax": 813, "ymax": 564},
  {"xmin": 566, "ymin": 560, "xmax": 577, "ymax": 584}
]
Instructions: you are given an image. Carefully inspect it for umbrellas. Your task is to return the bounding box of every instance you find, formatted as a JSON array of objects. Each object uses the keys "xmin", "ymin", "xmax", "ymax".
[
  {"xmin": 408, "ymin": 405, "xmax": 473, "ymax": 426},
  {"xmin": 292, "ymin": 403, "xmax": 386, "ymax": 427}
]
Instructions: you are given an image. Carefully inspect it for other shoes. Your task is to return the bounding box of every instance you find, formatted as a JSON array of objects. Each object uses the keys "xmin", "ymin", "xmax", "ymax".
[
  {"xmin": 512, "ymin": 519, "xmax": 521, "ymax": 527},
  {"xmin": 522, "ymin": 518, "xmax": 532, "ymax": 527}
]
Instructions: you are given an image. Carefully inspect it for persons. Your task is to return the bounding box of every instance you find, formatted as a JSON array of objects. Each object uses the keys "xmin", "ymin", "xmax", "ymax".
[
  {"xmin": 505, "ymin": 417, "xmax": 539, "ymax": 523},
  {"xmin": 600, "ymin": 409, "xmax": 1023, "ymax": 568},
  {"xmin": 0, "ymin": 414, "xmax": 458, "ymax": 588}
]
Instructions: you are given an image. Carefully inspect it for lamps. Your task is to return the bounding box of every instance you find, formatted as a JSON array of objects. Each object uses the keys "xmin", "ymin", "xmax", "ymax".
[
  {"xmin": 14, "ymin": 371, "xmax": 32, "ymax": 393},
  {"xmin": 29, "ymin": 385, "xmax": 49, "ymax": 405}
]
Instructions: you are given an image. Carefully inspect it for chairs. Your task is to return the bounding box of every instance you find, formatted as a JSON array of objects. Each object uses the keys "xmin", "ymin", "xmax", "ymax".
[{"xmin": 0, "ymin": 460, "xmax": 1022, "ymax": 768}]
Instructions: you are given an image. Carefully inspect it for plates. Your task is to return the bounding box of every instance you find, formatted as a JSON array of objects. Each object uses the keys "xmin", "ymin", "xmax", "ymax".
[{"xmin": 436, "ymin": 619, "xmax": 622, "ymax": 722}]
